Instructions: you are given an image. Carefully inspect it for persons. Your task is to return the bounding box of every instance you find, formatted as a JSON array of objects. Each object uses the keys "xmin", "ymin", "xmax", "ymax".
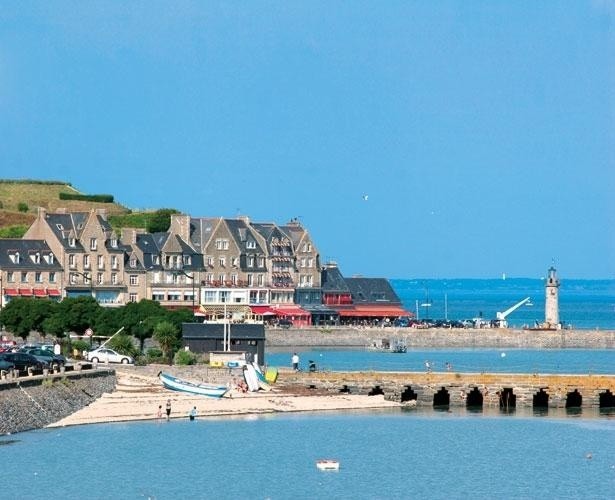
[
  {"xmin": 189, "ymin": 406, "xmax": 196, "ymax": 421},
  {"xmin": 165, "ymin": 399, "xmax": 172, "ymax": 421},
  {"xmin": 291, "ymin": 352, "xmax": 299, "ymax": 371},
  {"xmin": 156, "ymin": 406, "xmax": 162, "ymax": 417},
  {"xmin": 53, "ymin": 341, "xmax": 60, "ymax": 355}
]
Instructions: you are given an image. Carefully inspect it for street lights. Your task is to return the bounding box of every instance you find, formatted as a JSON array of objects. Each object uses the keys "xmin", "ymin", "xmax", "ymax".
[
  {"xmin": 175, "ymin": 269, "xmax": 194, "ymax": 313},
  {"xmin": 72, "ymin": 272, "xmax": 92, "ymax": 298}
]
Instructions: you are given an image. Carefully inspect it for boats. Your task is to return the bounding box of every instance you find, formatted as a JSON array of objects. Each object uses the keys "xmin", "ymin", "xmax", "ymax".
[
  {"xmin": 316, "ymin": 459, "xmax": 341, "ymax": 471},
  {"xmin": 421, "ymin": 303, "xmax": 433, "ymax": 307},
  {"xmin": 228, "ymin": 362, "xmax": 239, "ymax": 368},
  {"xmin": 365, "ymin": 336, "xmax": 407, "ymax": 353},
  {"xmin": 210, "ymin": 361, "xmax": 224, "ymax": 367},
  {"xmin": 157, "ymin": 371, "xmax": 231, "ymax": 398},
  {"xmin": 245, "ymin": 360, "xmax": 279, "ymax": 393}
]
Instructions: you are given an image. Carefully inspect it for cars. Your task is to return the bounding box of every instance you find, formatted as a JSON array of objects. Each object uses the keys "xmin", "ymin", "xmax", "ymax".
[
  {"xmin": 85, "ymin": 348, "xmax": 137, "ymax": 365},
  {"xmin": 0, "ymin": 331, "xmax": 68, "ymax": 376},
  {"xmin": 378, "ymin": 318, "xmax": 508, "ymax": 330},
  {"xmin": 263, "ymin": 319, "xmax": 293, "ymax": 330}
]
